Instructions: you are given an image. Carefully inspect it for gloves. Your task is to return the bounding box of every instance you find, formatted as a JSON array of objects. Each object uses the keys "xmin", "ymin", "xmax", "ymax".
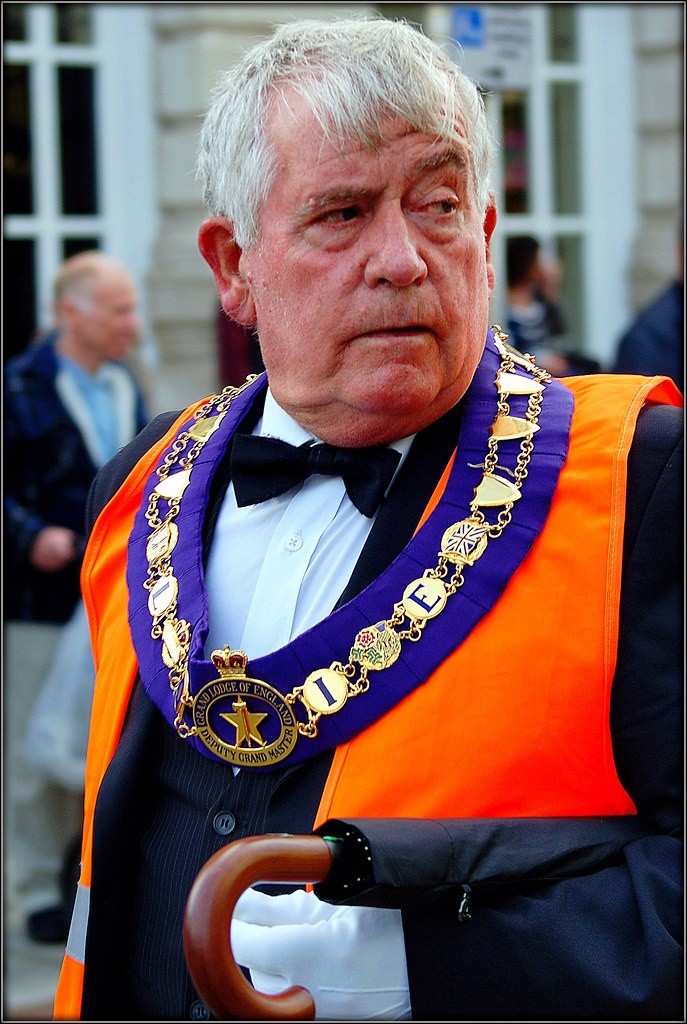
[{"xmin": 230, "ymin": 887, "xmax": 412, "ymax": 1021}]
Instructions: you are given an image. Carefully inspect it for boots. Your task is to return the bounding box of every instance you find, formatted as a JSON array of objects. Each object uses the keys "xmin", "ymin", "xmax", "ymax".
[{"xmin": 27, "ymin": 832, "xmax": 82, "ymax": 945}]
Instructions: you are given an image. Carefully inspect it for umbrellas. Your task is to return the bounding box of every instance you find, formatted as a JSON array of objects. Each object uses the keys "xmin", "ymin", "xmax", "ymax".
[{"xmin": 181, "ymin": 815, "xmax": 687, "ymax": 1021}]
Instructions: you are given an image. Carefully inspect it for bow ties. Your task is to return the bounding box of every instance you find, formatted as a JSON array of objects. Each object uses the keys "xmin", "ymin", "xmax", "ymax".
[{"xmin": 231, "ymin": 433, "xmax": 403, "ymax": 518}]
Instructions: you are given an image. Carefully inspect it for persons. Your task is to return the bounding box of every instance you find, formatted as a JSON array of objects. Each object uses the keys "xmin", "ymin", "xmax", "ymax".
[
  {"xmin": 504, "ymin": 231, "xmax": 600, "ymax": 378},
  {"xmin": 4, "ymin": 251, "xmax": 152, "ymax": 950},
  {"xmin": 614, "ymin": 200, "xmax": 684, "ymax": 391},
  {"xmin": 55, "ymin": 21, "xmax": 684, "ymax": 1021}
]
